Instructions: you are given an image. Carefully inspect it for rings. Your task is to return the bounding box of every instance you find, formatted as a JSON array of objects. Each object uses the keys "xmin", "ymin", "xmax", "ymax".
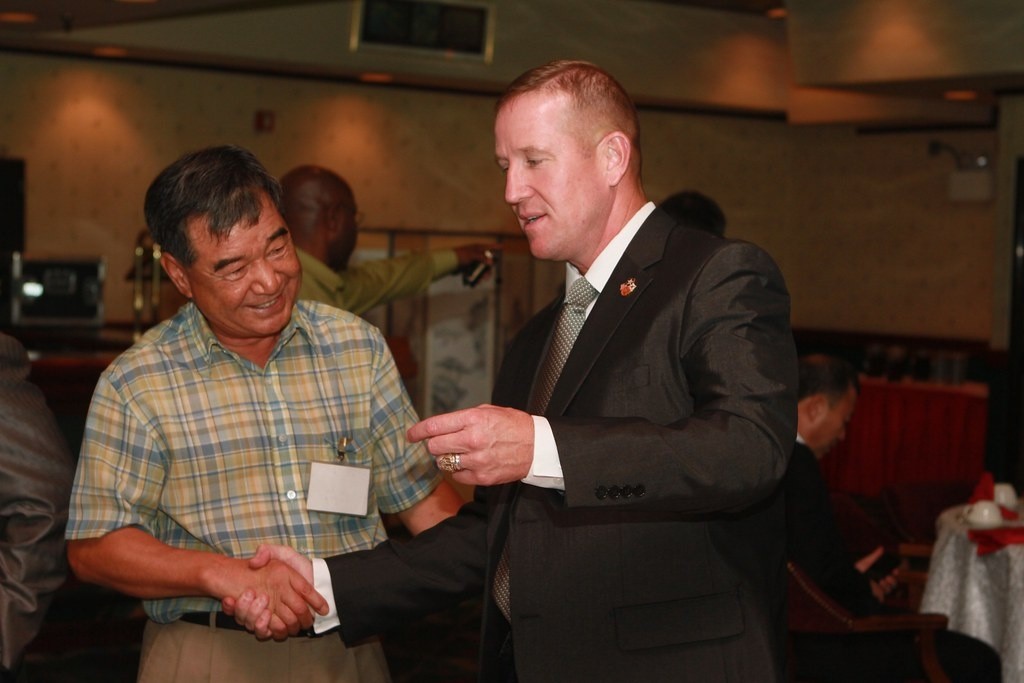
[{"xmin": 439, "ymin": 454, "xmax": 460, "ymax": 473}]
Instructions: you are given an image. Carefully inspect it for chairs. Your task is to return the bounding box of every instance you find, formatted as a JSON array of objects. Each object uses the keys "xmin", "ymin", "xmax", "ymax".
[{"xmin": 787, "ymin": 482, "xmax": 953, "ymax": 682}]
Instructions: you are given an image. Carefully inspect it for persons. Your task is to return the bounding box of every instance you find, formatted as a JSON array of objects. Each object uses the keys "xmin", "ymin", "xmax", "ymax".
[
  {"xmin": 65, "ymin": 143, "xmax": 466, "ymax": 683},
  {"xmin": 0, "ymin": 331, "xmax": 77, "ymax": 683},
  {"xmin": 221, "ymin": 59, "xmax": 800, "ymax": 682},
  {"xmin": 662, "ymin": 190, "xmax": 726, "ymax": 238},
  {"xmin": 778, "ymin": 354, "xmax": 1003, "ymax": 682},
  {"xmin": 276, "ymin": 164, "xmax": 505, "ymax": 323}
]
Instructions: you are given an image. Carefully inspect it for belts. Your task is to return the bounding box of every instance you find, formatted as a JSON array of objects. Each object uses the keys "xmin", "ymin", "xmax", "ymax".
[{"xmin": 180, "ymin": 611, "xmax": 325, "ymax": 638}]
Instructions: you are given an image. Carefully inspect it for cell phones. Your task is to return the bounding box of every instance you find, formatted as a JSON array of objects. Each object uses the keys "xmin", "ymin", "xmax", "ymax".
[{"xmin": 868, "ymin": 551, "xmax": 901, "ymax": 580}]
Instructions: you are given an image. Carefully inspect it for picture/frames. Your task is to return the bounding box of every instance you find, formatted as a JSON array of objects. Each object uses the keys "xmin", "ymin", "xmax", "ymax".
[{"xmin": 14, "ymin": 252, "xmax": 105, "ymax": 326}]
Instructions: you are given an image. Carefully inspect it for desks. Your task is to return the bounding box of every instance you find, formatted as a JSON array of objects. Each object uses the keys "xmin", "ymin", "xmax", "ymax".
[{"xmin": 923, "ymin": 500, "xmax": 1024, "ymax": 683}]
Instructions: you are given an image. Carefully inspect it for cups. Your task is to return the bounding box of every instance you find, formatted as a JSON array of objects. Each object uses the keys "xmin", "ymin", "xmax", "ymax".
[
  {"xmin": 964, "ymin": 501, "xmax": 1002, "ymax": 529},
  {"xmin": 993, "ymin": 484, "xmax": 1016, "ymax": 512}
]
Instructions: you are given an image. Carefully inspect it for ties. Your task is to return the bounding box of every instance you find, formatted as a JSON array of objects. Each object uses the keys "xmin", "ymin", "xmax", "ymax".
[{"xmin": 492, "ymin": 277, "xmax": 601, "ymax": 624}]
